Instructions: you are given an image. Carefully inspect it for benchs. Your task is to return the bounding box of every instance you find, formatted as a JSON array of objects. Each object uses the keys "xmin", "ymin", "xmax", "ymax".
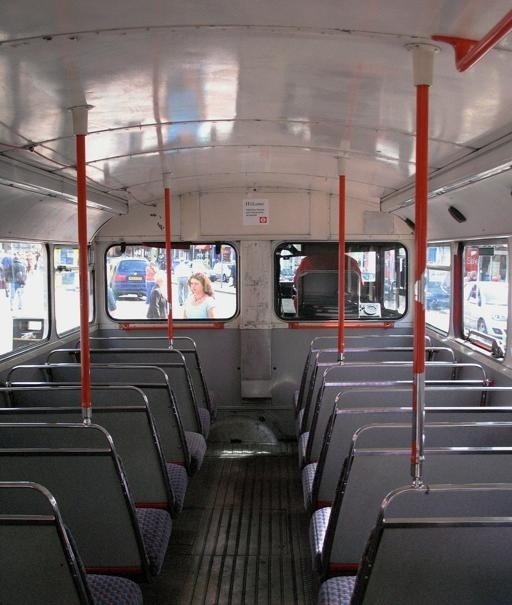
[
  {"xmin": 1, "ymin": 336, "xmax": 217, "ymax": 605},
  {"xmin": 292, "ymin": 332, "xmax": 511, "ymax": 605}
]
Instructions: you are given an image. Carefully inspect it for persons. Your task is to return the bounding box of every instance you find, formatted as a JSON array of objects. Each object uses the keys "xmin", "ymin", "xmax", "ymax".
[
  {"xmin": 293, "ymin": 254, "xmax": 365, "ymax": 312},
  {"xmin": 0, "ymin": 248, "xmax": 43, "ymax": 313},
  {"xmin": 145, "ymin": 260, "xmax": 158, "ymax": 306},
  {"xmin": 181, "ymin": 271, "xmax": 217, "ymax": 319},
  {"xmin": 228, "ymin": 259, "xmax": 236, "ymax": 289},
  {"xmin": 146, "ymin": 269, "xmax": 168, "ymax": 318},
  {"xmin": 174, "ymin": 257, "xmax": 190, "ymax": 307}
]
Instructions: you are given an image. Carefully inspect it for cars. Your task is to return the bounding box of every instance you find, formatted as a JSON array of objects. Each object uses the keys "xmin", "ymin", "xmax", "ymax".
[
  {"xmin": 278, "ymin": 300, "xmax": 452, "ymax": 310},
  {"xmin": 157, "ymin": 257, "xmax": 236, "ymax": 287},
  {"xmin": 463, "ymin": 280, "xmax": 509, "ymax": 340},
  {"xmin": 108, "ymin": 255, "xmax": 153, "ymax": 300}
]
